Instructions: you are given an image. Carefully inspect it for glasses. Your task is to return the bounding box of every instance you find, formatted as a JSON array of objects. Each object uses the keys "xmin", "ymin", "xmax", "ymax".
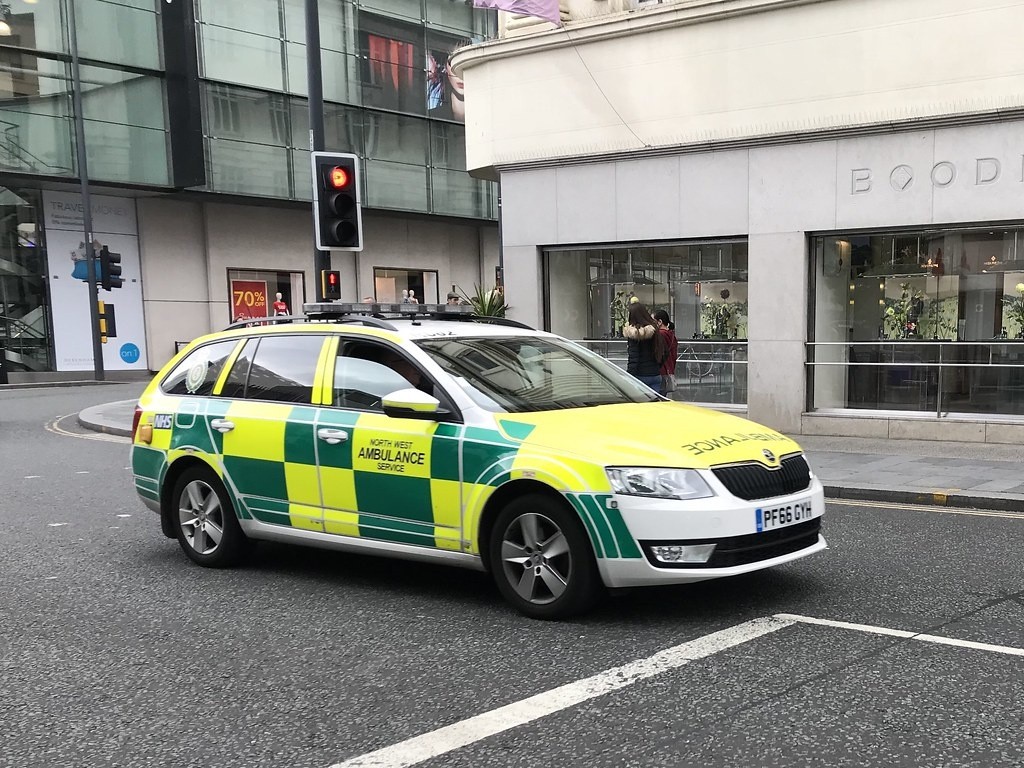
[{"xmin": 452, "ymin": 298, "xmax": 459, "ymax": 301}]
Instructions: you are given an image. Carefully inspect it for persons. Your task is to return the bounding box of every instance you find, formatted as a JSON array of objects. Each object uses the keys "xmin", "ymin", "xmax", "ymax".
[
  {"xmin": 428, "ymin": 37, "xmax": 472, "ymax": 122},
  {"xmin": 447, "ymin": 292, "xmax": 459, "ymax": 305},
  {"xmin": 408, "ymin": 290, "xmax": 419, "ymax": 304},
  {"xmin": 654, "ymin": 310, "xmax": 678, "ymax": 397},
  {"xmin": 623, "ymin": 303, "xmax": 668, "ymax": 393},
  {"xmin": 399, "ymin": 290, "xmax": 412, "ymax": 317},
  {"xmin": 272, "ymin": 292, "xmax": 289, "ymax": 324}
]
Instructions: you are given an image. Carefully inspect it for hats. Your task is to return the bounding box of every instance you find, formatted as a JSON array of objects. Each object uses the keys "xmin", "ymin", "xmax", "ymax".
[{"xmin": 447, "ymin": 291, "xmax": 459, "ymax": 299}]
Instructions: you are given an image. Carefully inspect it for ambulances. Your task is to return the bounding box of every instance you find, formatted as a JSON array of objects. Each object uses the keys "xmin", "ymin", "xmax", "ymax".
[{"xmin": 128, "ymin": 302, "xmax": 828, "ymax": 620}]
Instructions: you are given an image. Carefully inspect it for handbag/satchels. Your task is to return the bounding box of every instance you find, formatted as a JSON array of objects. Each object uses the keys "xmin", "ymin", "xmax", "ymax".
[{"xmin": 659, "ymin": 373, "xmax": 677, "ymax": 392}]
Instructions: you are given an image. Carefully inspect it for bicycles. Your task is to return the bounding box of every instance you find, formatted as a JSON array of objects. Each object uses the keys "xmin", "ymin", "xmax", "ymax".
[{"xmin": 677, "ymin": 332, "xmax": 713, "ymax": 377}]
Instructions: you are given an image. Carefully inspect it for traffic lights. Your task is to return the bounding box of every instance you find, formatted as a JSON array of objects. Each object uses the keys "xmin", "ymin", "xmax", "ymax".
[
  {"xmin": 310, "ymin": 153, "xmax": 363, "ymax": 251},
  {"xmin": 99, "ymin": 245, "xmax": 123, "ymax": 290},
  {"xmin": 319, "ymin": 270, "xmax": 342, "ymax": 299}
]
